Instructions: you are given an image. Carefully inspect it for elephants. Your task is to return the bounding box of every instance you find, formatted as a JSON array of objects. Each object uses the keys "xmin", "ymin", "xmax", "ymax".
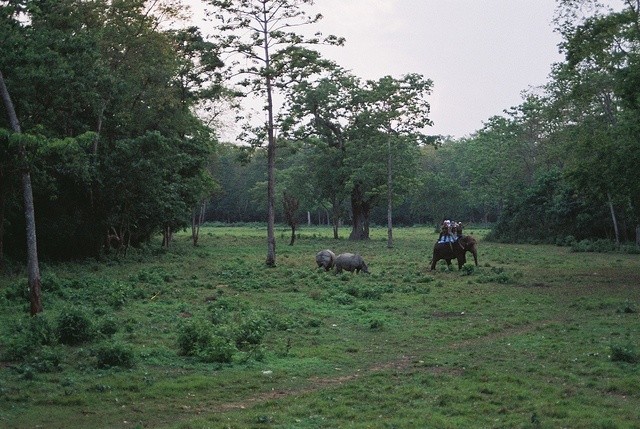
[
  {"xmin": 332, "ymin": 251, "xmax": 371, "ymax": 275},
  {"xmin": 429, "ymin": 234, "xmax": 478, "ymax": 270},
  {"xmin": 315, "ymin": 248, "xmax": 336, "ymax": 272}
]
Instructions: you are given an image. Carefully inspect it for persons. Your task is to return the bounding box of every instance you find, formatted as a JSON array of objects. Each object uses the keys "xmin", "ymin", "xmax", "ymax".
[{"xmin": 439, "ymin": 220, "xmax": 464, "ymax": 241}]
